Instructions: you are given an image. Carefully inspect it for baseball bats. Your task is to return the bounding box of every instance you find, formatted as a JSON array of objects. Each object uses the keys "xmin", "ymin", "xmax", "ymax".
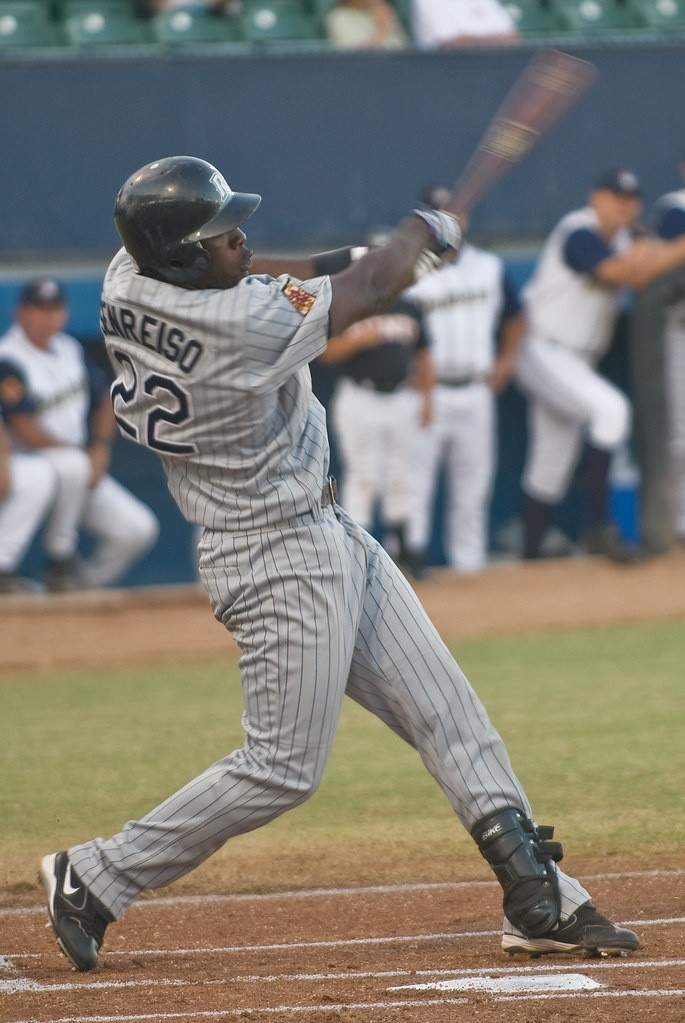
[{"xmin": 445, "ymin": 44, "xmax": 600, "ymax": 223}]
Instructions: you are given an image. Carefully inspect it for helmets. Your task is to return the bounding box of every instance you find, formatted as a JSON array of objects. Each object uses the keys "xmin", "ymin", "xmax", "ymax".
[{"xmin": 114, "ymin": 156, "xmax": 261, "ymax": 286}]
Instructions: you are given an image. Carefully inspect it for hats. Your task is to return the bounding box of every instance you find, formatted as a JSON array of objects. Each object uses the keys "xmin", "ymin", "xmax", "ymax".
[
  {"xmin": 598, "ymin": 167, "xmax": 641, "ymax": 196},
  {"xmin": 23, "ymin": 281, "xmax": 65, "ymax": 310},
  {"xmin": 424, "ymin": 188, "xmax": 450, "ymax": 209}
]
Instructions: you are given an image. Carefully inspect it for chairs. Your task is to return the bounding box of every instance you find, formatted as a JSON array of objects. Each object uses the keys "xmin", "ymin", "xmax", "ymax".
[{"xmin": 0, "ymin": 0, "xmax": 685, "ymax": 49}]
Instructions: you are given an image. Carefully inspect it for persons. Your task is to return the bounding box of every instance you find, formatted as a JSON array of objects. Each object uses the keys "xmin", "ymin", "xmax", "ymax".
[
  {"xmin": 0, "ymin": 276, "xmax": 159, "ymax": 591},
  {"xmin": 137, "ymin": 0, "xmax": 235, "ymax": 19},
  {"xmin": 323, "ymin": 0, "xmax": 406, "ymax": 48},
  {"xmin": 405, "ymin": 183, "xmax": 528, "ymax": 575},
  {"xmin": 320, "ymin": 222, "xmax": 433, "ymax": 566},
  {"xmin": 38, "ymin": 155, "xmax": 639, "ymax": 973},
  {"xmin": 654, "ymin": 184, "xmax": 685, "ymax": 547},
  {"xmin": 409, "ymin": 0, "xmax": 519, "ymax": 49},
  {"xmin": 515, "ymin": 172, "xmax": 665, "ymax": 560}
]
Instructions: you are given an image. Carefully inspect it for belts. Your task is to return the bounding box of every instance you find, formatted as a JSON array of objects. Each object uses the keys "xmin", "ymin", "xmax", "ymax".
[
  {"xmin": 437, "ymin": 378, "xmax": 472, "ymax": 388},
  {"xmin": 296, "ymin": 475, "xmax": 337, "ymax": 515}
]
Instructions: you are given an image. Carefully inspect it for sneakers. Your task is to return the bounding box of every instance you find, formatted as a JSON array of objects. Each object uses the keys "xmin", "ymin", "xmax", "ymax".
[
  {"xmin": 501, "ymin": 899, "xmax": 640, "ymax": 959},
  {"xmin": 38, "ymin": 850, "xmax": 114, "ymax": 972}
]
